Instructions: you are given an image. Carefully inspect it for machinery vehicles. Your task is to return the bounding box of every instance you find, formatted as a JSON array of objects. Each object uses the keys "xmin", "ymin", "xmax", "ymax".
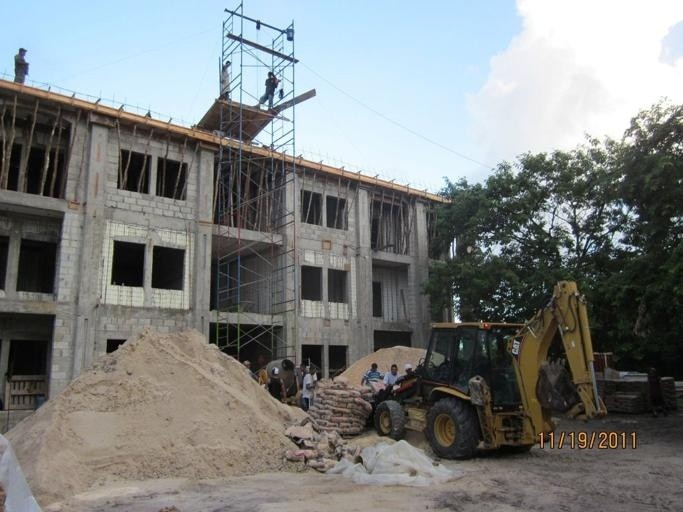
[{"xmin": 369, "ymin": 278, "xmax": 612, "ymax": 462}]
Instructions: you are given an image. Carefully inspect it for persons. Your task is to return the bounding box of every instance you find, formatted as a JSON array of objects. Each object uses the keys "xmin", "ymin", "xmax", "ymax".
[
  {"xmin": 11, "ymin": 48, "xmax": 29, "ymax": 86},
  {"xmin": 219, "ymin": 61, "xmax": 234, "ymax": 100},
  {"xmin": 253, "ymin": 71, "xmax": 279, "ymax": 112},
  {"xmin": 648, "ymin": 366, "xmax": 668, "ymax": 418},
  {"xmin": 239, "ymin": 358, "xmax": 414, "ymax": 414}
]
